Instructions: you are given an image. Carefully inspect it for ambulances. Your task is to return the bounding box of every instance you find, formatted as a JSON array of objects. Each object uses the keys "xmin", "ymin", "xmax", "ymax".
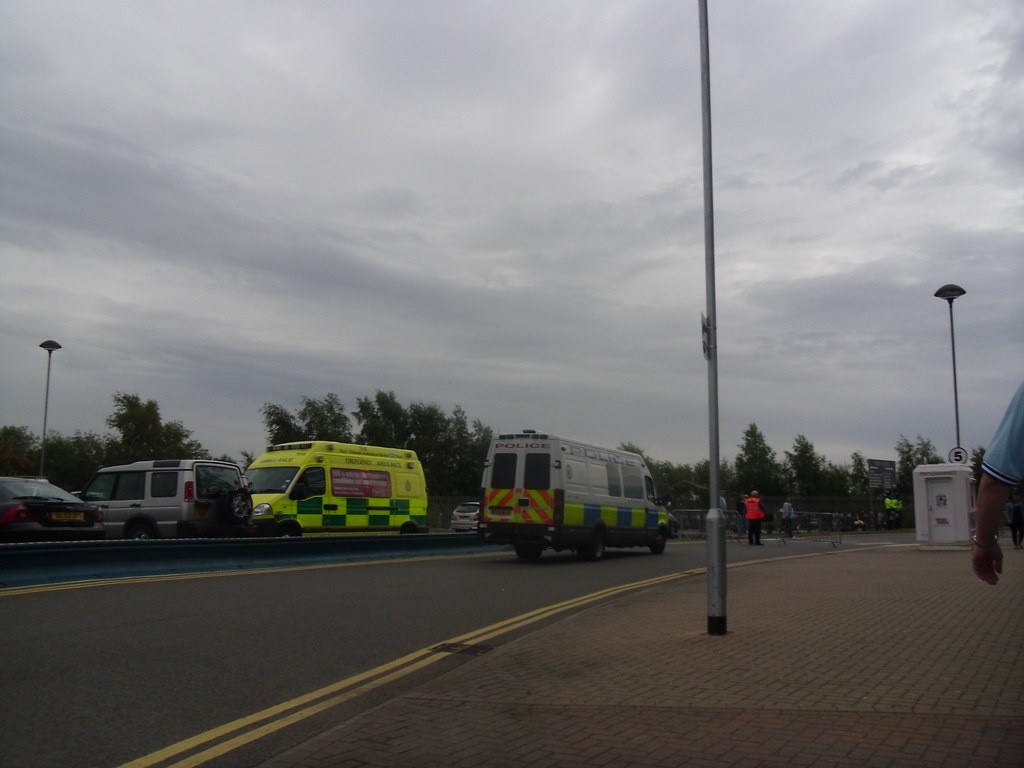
[{"xmin": 243, "ymin": 441, "xmax": 428, "ymax": 537}]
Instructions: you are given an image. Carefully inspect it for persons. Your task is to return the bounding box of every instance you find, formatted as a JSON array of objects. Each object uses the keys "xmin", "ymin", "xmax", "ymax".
[
  {"xmin": 737, "ymin": 490, "xmax": 767, "ymax": 546},
  {"xmin": 668, "ymin": 512, "xmax": 679, "ymax": 540},
  {"xmin": 1002, "ymin": 493, "xmax": 1024, "ymax": 549},
  {"xmin": 708, "ymin": 489, "xmax": 726, "ymax": 511},
  {"xmin": 837, "ymin": 509, "xmax": 897, "ymax": 531},
  {"xmin": 779, "ymin": 496, "xmax": 796, "ymax": 538},
  {"xmin": 971, "ymin": 382, "xmax": 1024, "ymax": 586}
]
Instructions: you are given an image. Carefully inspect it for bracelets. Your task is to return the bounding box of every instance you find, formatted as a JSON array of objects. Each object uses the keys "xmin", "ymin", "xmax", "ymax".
[{"xmin": 972, "ymin": 529, "xmax": 998, "ymax": 547}]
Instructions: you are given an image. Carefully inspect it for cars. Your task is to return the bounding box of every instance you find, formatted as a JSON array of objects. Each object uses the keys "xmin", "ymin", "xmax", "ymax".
[
  {"xmin": 450, "ymin": 502, "xmax": 480, "ymax": 532},
  {"xmin": 0, "ymin": 476, "xmax": 105, "ymax": 544}
]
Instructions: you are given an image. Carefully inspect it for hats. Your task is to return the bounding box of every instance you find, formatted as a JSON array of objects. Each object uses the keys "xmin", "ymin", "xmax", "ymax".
[{"xmin": 751, "ymin": 491, "xmax": 759, "ymax": 498}]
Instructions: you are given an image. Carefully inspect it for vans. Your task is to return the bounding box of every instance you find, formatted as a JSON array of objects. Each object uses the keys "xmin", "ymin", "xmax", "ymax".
[{"xmin": 78, "ymin": 459, "xmax": 254, "ymax": 540}]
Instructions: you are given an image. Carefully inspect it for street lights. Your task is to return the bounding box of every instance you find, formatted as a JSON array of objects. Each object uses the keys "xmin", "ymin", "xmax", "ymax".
[
  {"xmin": 934, "ymin": 284, "xmax": 967, "ymax": 447},
  {"xmin": 38, "ymin": 340, "xmax": 63, "ymax": 476}
]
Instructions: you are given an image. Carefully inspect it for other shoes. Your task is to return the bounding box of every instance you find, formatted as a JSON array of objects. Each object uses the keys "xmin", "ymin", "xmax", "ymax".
[{"xmin": 756, "ymin": 543, "xmax": 764, "ymax": 545}]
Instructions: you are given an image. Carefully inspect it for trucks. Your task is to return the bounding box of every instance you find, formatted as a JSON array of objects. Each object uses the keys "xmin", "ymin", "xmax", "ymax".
[{"xmin": 477, "ymin": 429, "xmax": 678, "ymax": 561}]
